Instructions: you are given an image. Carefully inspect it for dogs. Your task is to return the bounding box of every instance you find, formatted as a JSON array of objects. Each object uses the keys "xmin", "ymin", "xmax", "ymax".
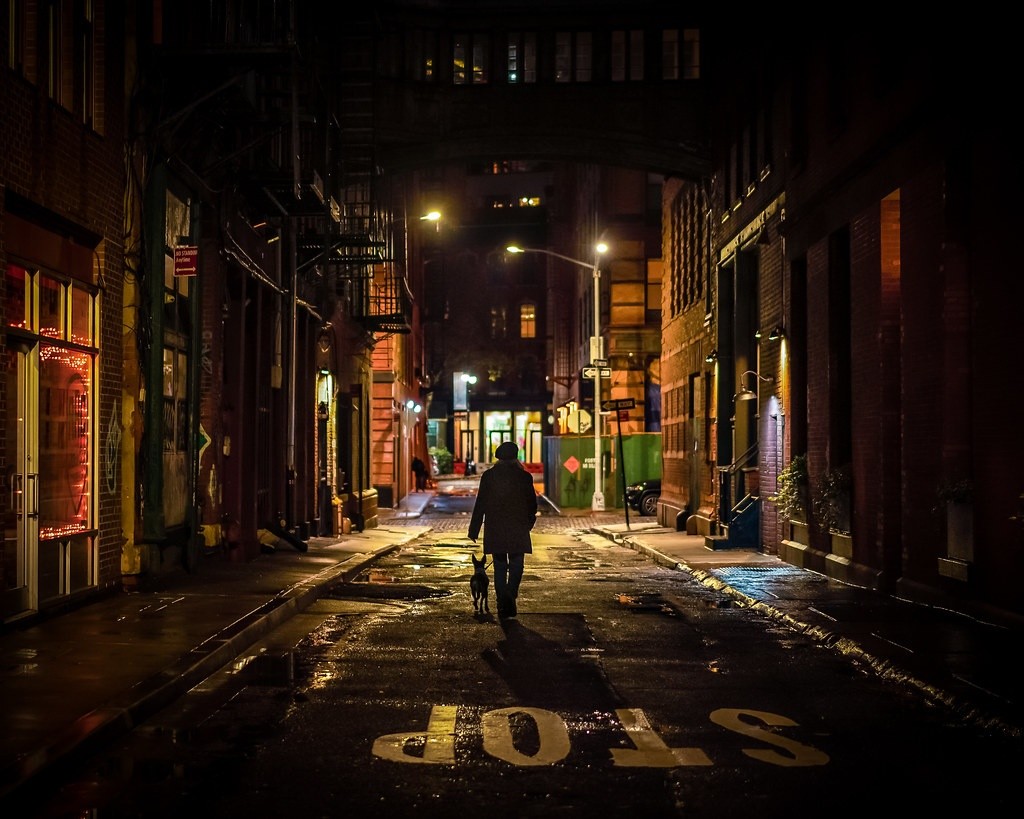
[{"xmin": 469, "ymin": 554, "xmax": 490, "ymax": 613}]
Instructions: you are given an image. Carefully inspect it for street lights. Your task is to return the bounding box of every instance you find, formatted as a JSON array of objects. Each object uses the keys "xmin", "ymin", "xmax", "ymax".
[
  {"xmin": 460, "ymin": 373, "xmax": 480, "ymax": 478},
  {"xmin": 508, "ymin": 244, "xmax": 608, "ymax": 511}
]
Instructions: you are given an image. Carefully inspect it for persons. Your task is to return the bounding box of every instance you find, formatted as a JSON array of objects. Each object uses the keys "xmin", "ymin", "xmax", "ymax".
[
  {"xmin": 412, "ymin": 457, "xmax": 425, "ymax": 492},
  {"xmin": 468, "ymin": 442, "xmax": 537, "ymax": 620}
]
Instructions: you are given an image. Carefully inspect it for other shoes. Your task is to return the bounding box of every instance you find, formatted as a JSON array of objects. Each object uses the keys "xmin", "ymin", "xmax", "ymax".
[
  {"xmin": 504, "ymin": 591, "xmax": 517, "ymax": 617},
  {"xmin": 498, "ymin": 608, "xmax": 508, "ymax": 618}
]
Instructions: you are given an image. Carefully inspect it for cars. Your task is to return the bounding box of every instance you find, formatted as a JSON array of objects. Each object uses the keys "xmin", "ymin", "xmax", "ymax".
[{"xmin": 624, "ymin": 478, "xmax": 663, "ymax": 517}]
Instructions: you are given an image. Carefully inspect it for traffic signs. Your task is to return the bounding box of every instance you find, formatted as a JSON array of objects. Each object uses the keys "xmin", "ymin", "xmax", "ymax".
[
  {"xmin": 599, "ymin": 397, "xmax": 635, "ymax": 413},
  {"xmin": 173, "ymin": 246, "xmax": 198, "ymax": 278},
  {"xmin": 581, "ymin": 367, "xmax": 612, "ymax": 379}
]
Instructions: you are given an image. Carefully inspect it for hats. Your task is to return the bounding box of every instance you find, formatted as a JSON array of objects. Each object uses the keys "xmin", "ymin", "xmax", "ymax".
[{"xmin": 495, "ymin": 442, "xmax": 518, "ymax": 459}]
